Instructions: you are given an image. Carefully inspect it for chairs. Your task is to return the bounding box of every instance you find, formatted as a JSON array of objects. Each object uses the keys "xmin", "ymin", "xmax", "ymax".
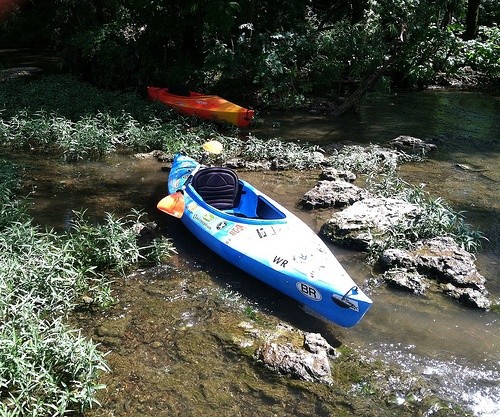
[
  {"xmin": 166, "ymin": 77, "xmax": 191, "ymax": 97},
  {"xmin": 191, "ymin": 167, "xmax": 239, "ymax": 210}
]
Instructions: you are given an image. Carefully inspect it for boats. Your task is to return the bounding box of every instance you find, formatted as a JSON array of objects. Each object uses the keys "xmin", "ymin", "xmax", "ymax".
[
  {"xmin": 168, "ymin": 154, "xmax": 373, "ymax": 328},
  {"xmin": 148, "ymin": 85, "xmax": 255, "ymax": 127}
]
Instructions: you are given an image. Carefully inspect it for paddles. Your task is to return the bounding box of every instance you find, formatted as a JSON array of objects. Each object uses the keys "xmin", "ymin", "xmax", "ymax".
[{"xmin": 157, "ymin": 152, "xmax": 209, "ymax": 219}]
